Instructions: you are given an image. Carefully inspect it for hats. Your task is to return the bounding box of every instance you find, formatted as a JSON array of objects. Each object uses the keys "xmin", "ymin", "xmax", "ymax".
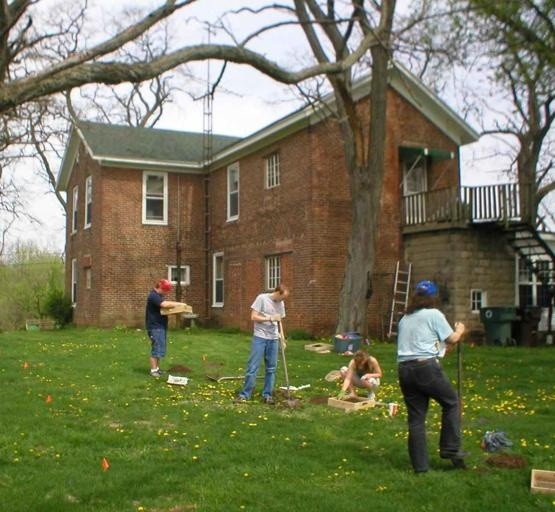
[
  {"xmin": 159, "ymin": 279, "xmax": 172, "ymax": 291},
  {"xmin": 416, "ymin": 280, "xmax": 438, "ymax": 295}
]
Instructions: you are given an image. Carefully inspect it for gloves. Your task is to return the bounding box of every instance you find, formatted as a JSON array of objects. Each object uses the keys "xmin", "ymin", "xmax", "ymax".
[
  {"xmin": 280, "ymin": 341, "xmax": 287, "ymax": 349},
  {"xmin": 270, "ymin": 314, "xmax": 281, "ymax": 322},
  {"xmin": 337, "ymin": 390, "xmax": 346, "ymax": 400},
  {"xmin": 176, "ymin": 303, "xmax": 187, "ymax": 310}
]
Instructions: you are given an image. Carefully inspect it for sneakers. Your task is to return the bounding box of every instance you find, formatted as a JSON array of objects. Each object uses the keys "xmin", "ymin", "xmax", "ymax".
[
  {"xmin": 368, "ymin": 392, "xmax": 375, "ymax": 400},
  {"xmin": 150, "ymin": 371, "xmax": 162, "ymax": 377},
  {"xmin": 237, "ymin": 396, "xmax": 245, "ymax": 400},
  {"xmin": 264, "ymin": 397, "xmax": 275, "ymax": 405},
  {"xmin": 440, "ymin": 449, "xmax": 467, "ymax": 459}
]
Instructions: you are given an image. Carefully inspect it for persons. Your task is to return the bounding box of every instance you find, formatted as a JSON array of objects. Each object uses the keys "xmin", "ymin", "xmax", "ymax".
[
  {"xmin": 236, "ymin": 283, "xmax": 291, "ymax": 404},
  {"xmin": 145, "ymin": 278, "xmax": 188, "ymax": 378},
  {"xmin": 335, "ymin": 349, "xmax": 384, "ymax": 401},
  {"xmin": 396, "ymin": 279, "xmax": 471, "ymax": 473}
]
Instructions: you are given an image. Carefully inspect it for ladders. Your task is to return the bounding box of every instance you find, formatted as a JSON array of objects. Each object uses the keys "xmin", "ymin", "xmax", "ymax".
[{"xmin": 387, "ymin": 261, "xmax": 412, "ymax": 338}]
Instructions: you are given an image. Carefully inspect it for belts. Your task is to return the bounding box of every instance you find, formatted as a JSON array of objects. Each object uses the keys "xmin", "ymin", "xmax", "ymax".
[{"xmin": 400, "ymin": 360, "xmax": 417, "ymax": 365}]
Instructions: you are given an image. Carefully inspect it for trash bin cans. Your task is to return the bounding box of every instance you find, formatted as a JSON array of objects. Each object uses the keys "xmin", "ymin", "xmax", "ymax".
[
  {"xmin": 480, "ymin": 307, "xmax": 517, "ymax": 347},
  {"xmin": 517, "ymin": 306, "xmax": 543, "ymax": 347}
]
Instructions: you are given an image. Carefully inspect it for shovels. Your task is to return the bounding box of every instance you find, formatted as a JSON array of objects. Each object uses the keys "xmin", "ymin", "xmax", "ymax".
[
  {"xmin": 207, "ymin": 376, "xmax": 265, "ymax": 384},
  {"xmin": 450, "ymin": 341, "xmax": 466, "ymax": 470},
  {"xmin": 280, "ymin": 322, "xmax": 303, "ymax": 410}
]
